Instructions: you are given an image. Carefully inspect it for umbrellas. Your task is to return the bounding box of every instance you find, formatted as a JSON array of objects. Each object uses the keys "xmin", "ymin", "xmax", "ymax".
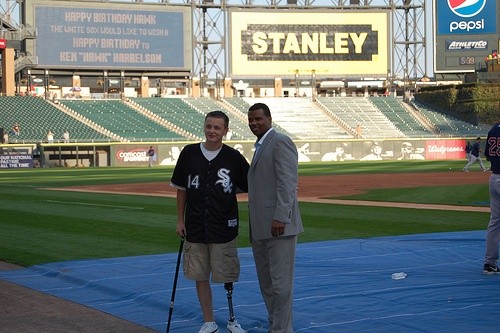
[{"xmin": 70, "ymin": 86, "xmax": 81, "ymax": 94}]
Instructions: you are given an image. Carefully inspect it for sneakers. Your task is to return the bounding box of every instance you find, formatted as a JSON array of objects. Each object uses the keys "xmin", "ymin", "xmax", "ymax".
[
  {"xmin": 198, "ymin": 322, "xmax": 219, "ymax": 333},
  {"xmin": 482, "ymin": 263, "xmax": 500, "ymax": 274},
  {"xmin": 227, "ymin": 319, "xmax": 248, "ymax": 333}
]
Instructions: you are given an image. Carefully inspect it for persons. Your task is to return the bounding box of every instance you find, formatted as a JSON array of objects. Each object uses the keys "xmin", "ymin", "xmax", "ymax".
[
  {"xmin": 63, "ymin": 129, "xmax": 70, "ymax": 142},
  {"xmin": 47, "ymin": 130, "xmax": 54, "ymax": 143},
  {"xmin": 355, "ymin": 124, "xmax": 361, "ymax": 139},
  {"xmin": 146, "ymin": 146, "xmax": 154, "ymax": 166},
  {"xmin": 3, "ymin": 132, "xmax": 9, "ymax": 143},
  {"xmin": 465, "ymin": 142, "xmax": 472, "ymax": 163},
  {"xmin": 13, "ymin": 122, "xmax": 20, "ymax": 136},
  {"xmin": 464, "ymin": 137, "xmax": 489, "ymax": 172},
  {"xmin": 248, "ymin": 103, "xmax": 304, "ymax": 333},
  {"xmin": 170, "ymin": 111, "xmax": 250, "ymax": 333},
  {"xmin": 483, "ymin": 122, "xmax": 500, "ymax": 275},
  {"xmin": 226, "ymin": 131, "xmax": 232, "ymax": 141}
]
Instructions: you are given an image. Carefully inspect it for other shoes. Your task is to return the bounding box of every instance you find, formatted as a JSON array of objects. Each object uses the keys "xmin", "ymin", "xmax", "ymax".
[
  {"xmin": 484, "ymin": 168, "xmax": 490, "ymax": 171},
  {"xmin": 464, "ymin": 168, "xmax": 469, "ymax": 172}
]
